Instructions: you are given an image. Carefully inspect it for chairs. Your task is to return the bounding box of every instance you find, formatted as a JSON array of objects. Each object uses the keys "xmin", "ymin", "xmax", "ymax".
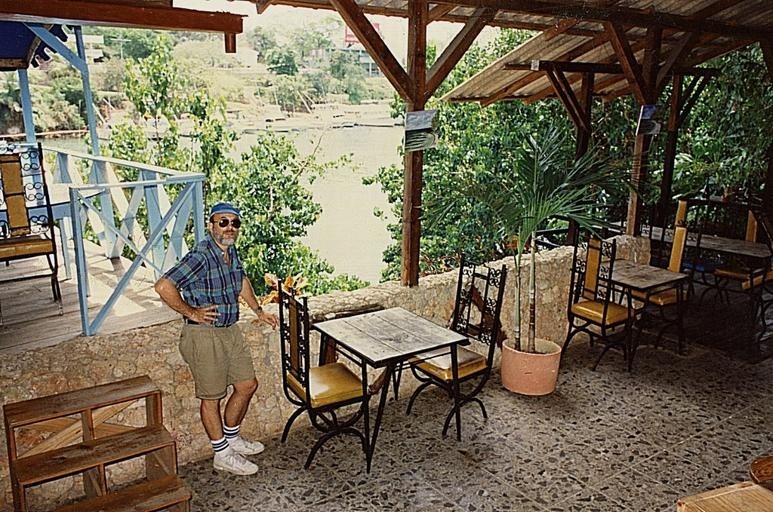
[
  {"xmin": 1, "ymin": 139, "xmax": 66, "ymax": 308},
  {"xmin": 560, "ymin": 228, "xmax": 635, "ymax": 372},
  {"xmin": 406, "ymin": 252, "xmax": 508, "ymax": 440},
  {"xmin": 276, "ymin": 278, "xmax": 373, "ymax": 474},
  {"xmin": 628, "ymin": 193, "xmax": 772, "ymax": 364}
]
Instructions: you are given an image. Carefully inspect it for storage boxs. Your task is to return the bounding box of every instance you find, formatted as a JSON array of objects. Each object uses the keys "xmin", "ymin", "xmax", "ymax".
[{"xmin": 676, "ymin": 481, "xmax": 773, "ymax": 512}]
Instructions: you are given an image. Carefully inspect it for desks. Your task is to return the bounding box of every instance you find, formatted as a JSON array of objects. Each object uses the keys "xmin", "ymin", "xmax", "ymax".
[
  {"xmin": 308, "ymin": 305, "xmax": 473, "ymax": 475},
  {"xmin": 1, "ymin": 183, "xmax": 106, "ymax": 279},
  {"xmin": 568, "ymin": 258, "xmax": 690, "ymax": 374}
]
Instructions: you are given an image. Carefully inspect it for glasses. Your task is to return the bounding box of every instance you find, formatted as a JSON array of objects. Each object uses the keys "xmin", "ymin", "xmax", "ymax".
[{"xmin": 213, "ymin": 218, "xmax": 241, "ymax": 228}]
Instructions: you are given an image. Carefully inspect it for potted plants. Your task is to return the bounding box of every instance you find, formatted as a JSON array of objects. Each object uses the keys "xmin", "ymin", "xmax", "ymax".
[{"xmin": 413, "ymin": 112, "xmax": 656, "ymax": 397}]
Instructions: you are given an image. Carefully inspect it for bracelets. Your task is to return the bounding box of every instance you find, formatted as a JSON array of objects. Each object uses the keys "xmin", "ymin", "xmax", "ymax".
[{"xmin": 256, "ymin": 307, "xmax": 262, "ymax": 315}]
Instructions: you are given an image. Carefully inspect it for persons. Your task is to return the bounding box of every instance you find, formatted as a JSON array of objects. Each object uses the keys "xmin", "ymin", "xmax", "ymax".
[{"xmin": 154, "ymin": 203, "xmax": 276, "ymax": 476}]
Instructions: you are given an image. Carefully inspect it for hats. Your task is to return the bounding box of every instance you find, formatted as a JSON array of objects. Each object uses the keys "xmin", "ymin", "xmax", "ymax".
[{"xmin": 209, "ymin": 202, "xmax": 240, "ymax": 217}]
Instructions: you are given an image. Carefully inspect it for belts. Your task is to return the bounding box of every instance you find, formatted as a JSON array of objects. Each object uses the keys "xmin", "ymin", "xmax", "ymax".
[{"xmin": 184, "ymin": 318, "xmax": 235, "ymax": 328}]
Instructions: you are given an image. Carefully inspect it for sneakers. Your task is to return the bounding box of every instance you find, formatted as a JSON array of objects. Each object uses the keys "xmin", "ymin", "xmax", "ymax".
[
  {"xmin": 213, "ymin": 448, "xmax": 259, "ymax": 476},
  {"xmin": 229, "ymin": 436, "xmax": 265, "ymax": 455}
]
